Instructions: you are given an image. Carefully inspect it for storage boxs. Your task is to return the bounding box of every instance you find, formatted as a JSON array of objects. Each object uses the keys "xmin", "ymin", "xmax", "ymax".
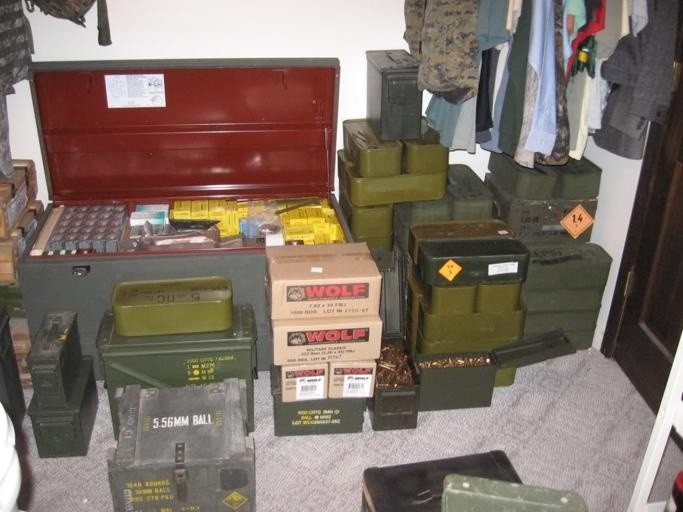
[{"xmin": 0, "ymin": 50, "xmax": 613, "ymax": 512}]
